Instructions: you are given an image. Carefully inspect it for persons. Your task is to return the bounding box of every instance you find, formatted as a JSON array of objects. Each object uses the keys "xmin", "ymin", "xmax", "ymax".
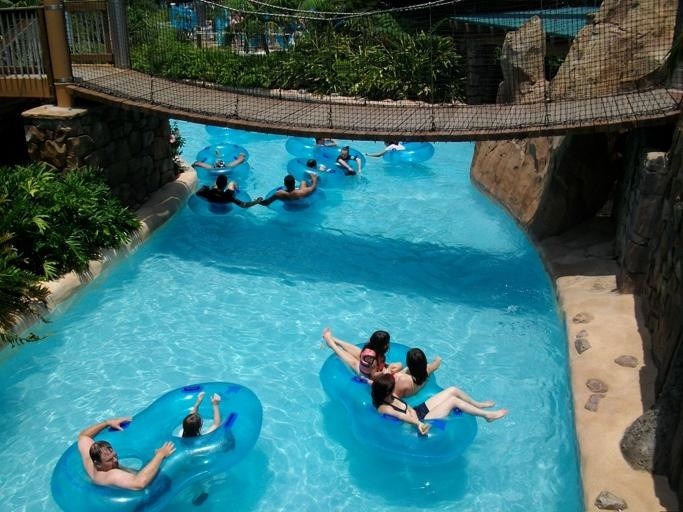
[
  {"xmin": 178, "ymin": 392, "xmax": 221, "ymax": 438},
  {"xmin": 76, "ymin": 417, "xmax": 176, "ymax": 491},
  {"xmin": 227, "ymin": 12, "xmax": 306, "ymax": 51},
  {"xmin": 306, "ymin": 137, "xmax": 406, "ymax": 174},
  {"xmin": 371, "ymin": 373, "xmax": 507, "ymax": 436},
  {"xmin": 259, "ymin": 173, "xmax": 317, "ymax": 206},
  {"xmin": 358, "ymin": 330, "xmax": 403, "ymax": 380},
  {"xmin": 192, "ymin": 154, "xmax": 244, "ymax": 168},
  {"xmin": 196, "ymin": 175, "xmax": 262, "ymax": 209},
  {"xmin": 321, "ymin": 326, "xmax": 441, "ymax": 397}
]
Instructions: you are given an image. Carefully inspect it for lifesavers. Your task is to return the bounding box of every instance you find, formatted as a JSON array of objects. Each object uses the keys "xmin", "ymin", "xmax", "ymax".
[
  {"xmin": 319, "ymin": 340, "xmax": 478, "ymax": 458},
  {"xmin": 189, "ymin": 134, "xmax": 436, "ymax": 213},
  {"xmin": 51, "ymin": 383, "xmax": 262, "ymax": 512}
]
